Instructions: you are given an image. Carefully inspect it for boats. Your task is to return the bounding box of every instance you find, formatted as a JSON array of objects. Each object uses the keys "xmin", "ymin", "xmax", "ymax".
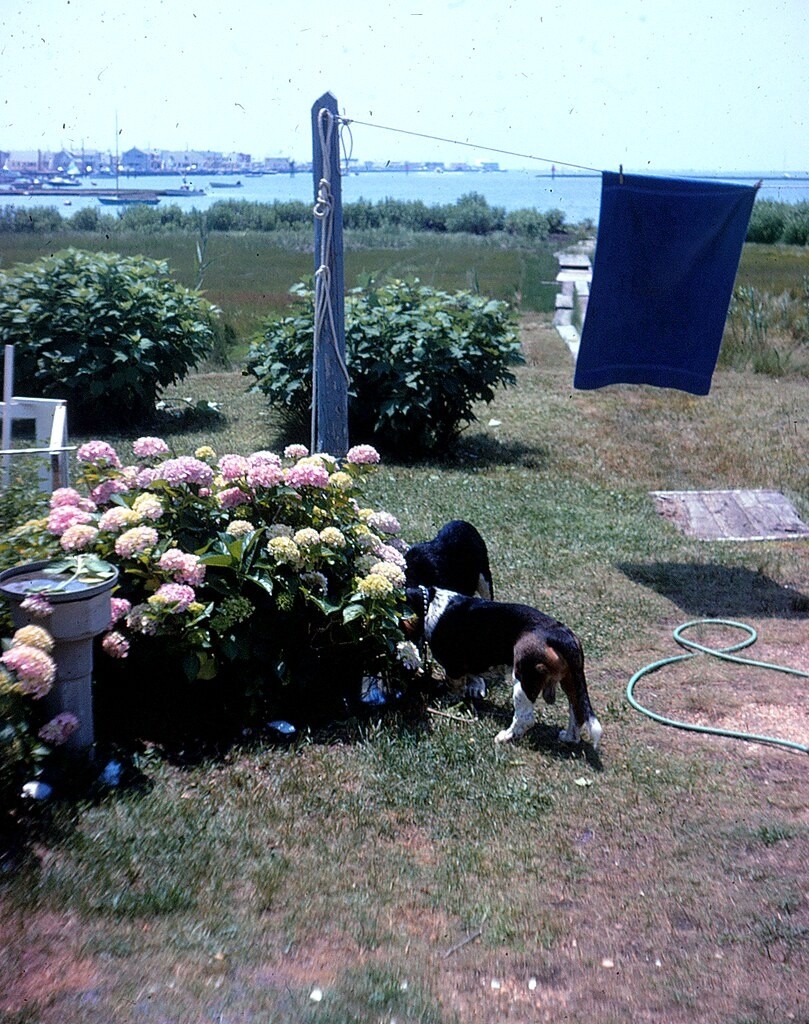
[
  {"xmin": 48, "ymin": 176, "xmax": 82, "ymax": 186},
  {"xmin": 164, "ymin": 188, "xmax": 209, "ymax": 198},
  {"xmin": 209, "ymin": 180, "xmax": 245, "ymax": 188},
  {"xmin": 11, "ymin": 176, "xmax": 44, "ymax": 189}
]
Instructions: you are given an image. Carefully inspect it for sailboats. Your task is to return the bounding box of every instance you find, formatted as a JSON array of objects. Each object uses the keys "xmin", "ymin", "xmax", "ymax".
[{"xmin": 96, "ymin": 110, "xmax": 162, "ymax": 205}]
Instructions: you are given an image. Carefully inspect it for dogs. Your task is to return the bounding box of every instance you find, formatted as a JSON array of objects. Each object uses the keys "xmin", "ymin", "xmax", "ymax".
[
  {"xmin": 402, "ymin": 586, "xmax": 603, "ymax": 753},
  {"xmin": 404, "ymin": 520, "xmax": 494, "ymax": 601}
]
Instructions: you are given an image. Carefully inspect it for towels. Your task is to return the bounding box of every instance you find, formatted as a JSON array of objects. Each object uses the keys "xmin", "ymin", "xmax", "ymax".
[{"xmin": 572, "ymin": 170, "xmax": 759, "ymax": 397}]
